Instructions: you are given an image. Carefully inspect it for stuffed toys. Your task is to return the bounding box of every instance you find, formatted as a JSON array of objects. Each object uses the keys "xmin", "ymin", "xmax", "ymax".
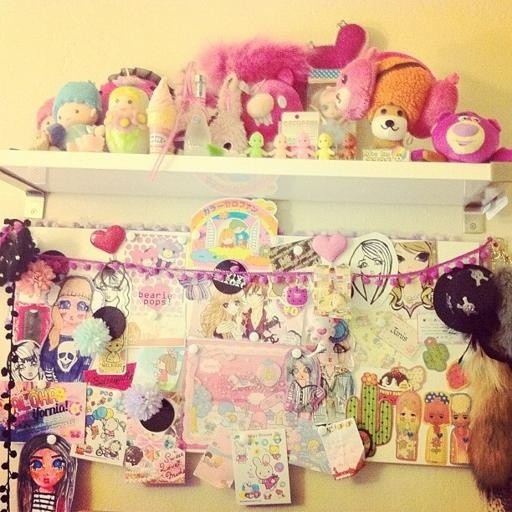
[
  {"xmin": 29, "ymin": 17, "xmax": 512, "ymax": 164},
  {"xmin": 29, "ymin": 17, "xmax": 511, "ymax": 165}
]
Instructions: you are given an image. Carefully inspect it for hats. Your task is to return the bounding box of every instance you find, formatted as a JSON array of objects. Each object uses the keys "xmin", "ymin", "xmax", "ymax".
[{"xmin": 432, "ymin": 263, "xmax": 501, "ymax": 336}]
